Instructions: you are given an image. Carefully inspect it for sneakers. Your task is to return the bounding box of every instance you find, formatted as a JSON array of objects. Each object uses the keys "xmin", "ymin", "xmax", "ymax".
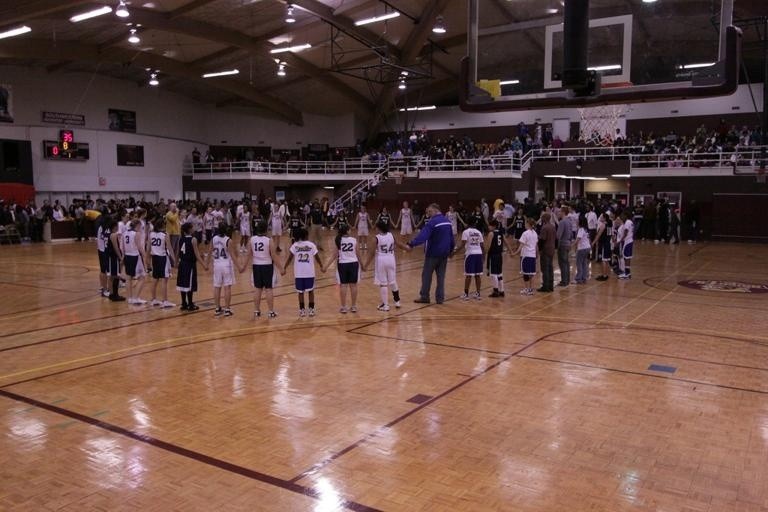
[
  {"xmin": 214, "ymin": 307, "xmax": 224, "ymax": 315},
  {"xmin": 414, "ymin": 296, "xmax": 430, "ymax": 303},
  {"xmin": 180, "ymin": 303, "xmax": 188, "ymax": 310},
  {"xmin": 377, "ymin": 303, "xmax": 389, "ymax": 311},
  {"xmin": 109, "ymin": 294, "xmax": 126, "ymax": 301},
  {"xmin": 98, "ymin": 288, "xmax": 111, "ymax": 296},
  {"xmin": 459, "ymin": 273, "xmax": 637, "ymax": 300},
  {"xmin": 298, "ymin": 308, "xmax": 316, "ymax": 317},
  {"xmin": 340, "ymin": 305, "xmax": 358, "ymax": 313},
  {"xmin": 254, "ymin": 311, "xmax": 261, "ymax": 320},
  {"xmin": 394, "ymin": 301, "xmax": 400, "ymax": 307},
  {"xmin": 151, "ymin": 298, "xmax": 162, "ymax": 306},
  {"xmin": 437, "ymin": 299, "xmax": 443, "ymax": 304},
  {"xmin": 225, "ymin": 309, "xmax": 234, "ymax": 316},
  {"xmin": 268, "ymin": 312, "xmax": 278, "ymax": 319},
  {"xmin": 188, "ymin": 306, "xmax": 199, "ymax": 310},
  {"xmin": 128, "ymin": 297, "xmax": 147, "ymax": 304},
  {"xmin": 162, "ymin": 300, "xmax": 176, "ymax": 308}
]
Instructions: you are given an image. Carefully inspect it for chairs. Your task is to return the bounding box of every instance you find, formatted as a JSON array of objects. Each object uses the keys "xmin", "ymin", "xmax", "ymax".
[
  {"xmin": 0, "ymin": 225, "xmax": 11, "ymax": 246},
  {"xmin": 5, "ymin": 223, "xmax": 22, "ymax": 246}
]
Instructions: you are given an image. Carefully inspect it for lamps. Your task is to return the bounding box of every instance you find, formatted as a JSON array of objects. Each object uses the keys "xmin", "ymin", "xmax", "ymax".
[
  {"xmin": 127, "ymin": 23, "xmax": 141, "ymax": 44},
  {"xmin": 432, "ymin": 15, "xmax": 446, "ymax": 34},
  {"xmin": 146, "ymin": 68, "xmax": 161, "ymax": 85},
  {"xmin": 203, "ymin": 68, "xmax": 240, "ymax": 78},
  {"xmin": 0, "ymin": 26, "xmax": 31, "ymax": 39},
  {"xmin": 275, "ymin": 59, "xmax": 287, "ymax": 76},
  {"xmin": 116, "ymin": 2, "xmax": 130, "ymax": 18},
  {"xmin": 355, "ymin": 12, "xmax": 401, "ymax": 26},
  {"xmin": 270, "ymin": 43, "xmax": 312, "ymax": 54},
  {"xmin": 398, "ymin": 71, "xmax": 408, "ymax": 90},
  {"xmin": 285, "ymin": 4, "xmax": 296, "ymax": 23},
  {"xmin": 70, "ymin": 6, "xmax": 112, "ymax": 22}
]
